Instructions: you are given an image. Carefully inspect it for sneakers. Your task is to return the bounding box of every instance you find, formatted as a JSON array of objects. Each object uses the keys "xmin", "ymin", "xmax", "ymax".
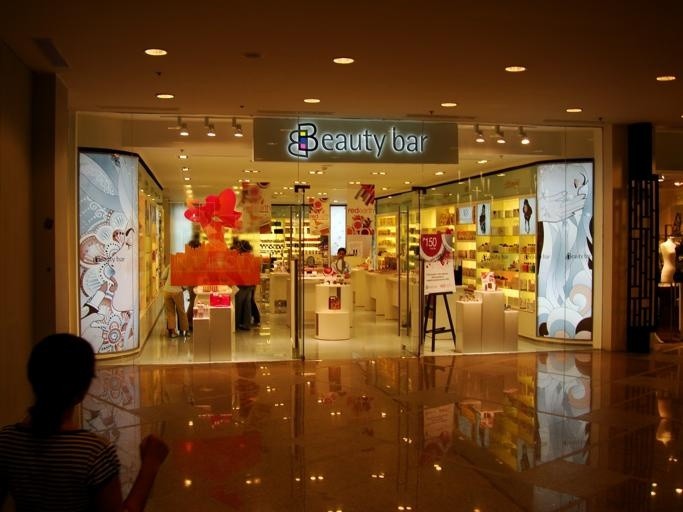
[
  {"xmin": 235, "ymin": 319, "xmax": 260, "ymax": 330},
  {"xmin": 167, "ymin": 326, "xmax": 193, "ymax": 339}
]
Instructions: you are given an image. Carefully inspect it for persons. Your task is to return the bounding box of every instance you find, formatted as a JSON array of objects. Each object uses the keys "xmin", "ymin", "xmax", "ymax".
[
  {"xmin": 0, "ymin": 331, "xmax": 171, "ymax": 512},
  {"xmin": 157, "ymin": 232, "xmax": 260, "ymax": 339},
  {"xmin": 330, "ymin": 246, "xmax": 351, "ymax": 276},
  {"xmin": 660, "ymin": 235, "xmax": 679, "ymax": 285},
  {"xmin": 655, "ymin": 395, "xmax": 673, "ymax": 445}
]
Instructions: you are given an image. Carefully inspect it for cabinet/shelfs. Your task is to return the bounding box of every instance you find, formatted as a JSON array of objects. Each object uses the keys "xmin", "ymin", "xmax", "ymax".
[
  {"xmin": 197, "ymin": 203, "xmax": 328, "ymax": 310},
  {"xmin": 372, "ymin": 193, "xmax": 537, "ymax": 344}
]
